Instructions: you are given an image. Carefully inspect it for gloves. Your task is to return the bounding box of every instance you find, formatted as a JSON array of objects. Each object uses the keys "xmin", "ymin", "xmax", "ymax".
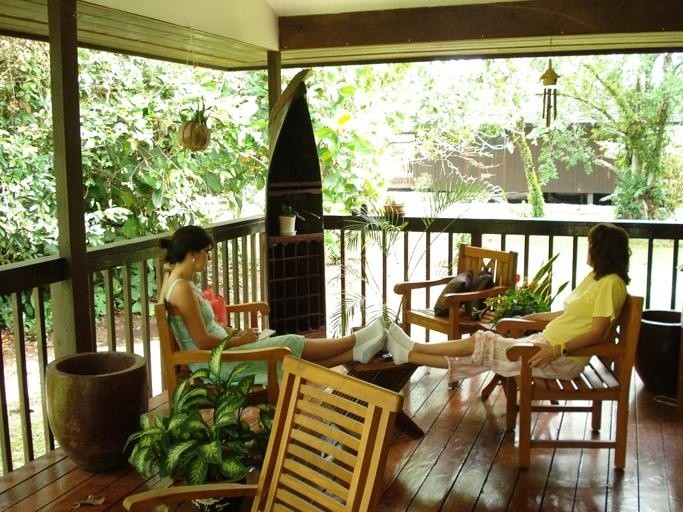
[{"xmin": 527, "ymin": 343, "xmax": 561, "ymax": 370}]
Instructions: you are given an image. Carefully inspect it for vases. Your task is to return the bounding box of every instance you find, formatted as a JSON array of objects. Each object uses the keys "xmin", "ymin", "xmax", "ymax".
[
  {"xmin": 45, "ymin": 352, "xmax": 149, "ymax": 473},
  {"xmin": 635, "ymin": 308, "xmax": 682, "ymax": 395}
]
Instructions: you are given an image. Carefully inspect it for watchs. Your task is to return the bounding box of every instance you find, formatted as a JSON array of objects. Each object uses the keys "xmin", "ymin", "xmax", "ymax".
[{"xmin": 560, "ymin": 343, "xmax": 569, "ymax": 357}]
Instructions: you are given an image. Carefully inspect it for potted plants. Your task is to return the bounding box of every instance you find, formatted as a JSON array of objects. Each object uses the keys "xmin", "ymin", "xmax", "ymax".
[
  {"xmin": 329, "ymin": 178, "xmax": 511, "ymax": 339},
  {"xmin": 121, "ymin": 328, "xmax": 282, "ymax": 512},
  {"xmin": 277, "ymin": 203, "xmax": 304, "ymax": 236}
]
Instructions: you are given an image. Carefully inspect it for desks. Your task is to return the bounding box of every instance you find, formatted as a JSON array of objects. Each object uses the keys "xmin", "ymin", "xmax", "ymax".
[{"xmin": 472, "ymin": 319, "xmax": 560, "ymax": 418}]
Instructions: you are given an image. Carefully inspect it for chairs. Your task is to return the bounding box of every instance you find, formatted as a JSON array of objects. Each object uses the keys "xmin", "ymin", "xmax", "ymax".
[
  {"xmin": 503, "ymin": 291, "xmax": 643, "ymax": 465},
  {"xmin": 393, "ymin": 244, "xmax": 519, "ymax": 391},
  {"xmin": 122, "ymin": 355, "xmax": 404, "ymax": 512}
]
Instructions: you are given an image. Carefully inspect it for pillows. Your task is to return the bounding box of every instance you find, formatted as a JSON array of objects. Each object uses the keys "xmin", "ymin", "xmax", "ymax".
[
  {"xmin": 434, "ymin": 270, "xmax": 473, "ymax": 316},
  {"xmin": 464, "ymin": 268, "xmax": 495, "ymax": 317}
]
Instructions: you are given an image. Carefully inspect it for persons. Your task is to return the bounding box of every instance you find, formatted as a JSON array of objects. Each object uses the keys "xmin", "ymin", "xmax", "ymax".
[
  {"xmin": 157, "ymin": 226, "xmax": 387, "ymax": 386},
  {"xmin": 385, "ymin": 224, "xmax": 632, "ymax": 381}
]
